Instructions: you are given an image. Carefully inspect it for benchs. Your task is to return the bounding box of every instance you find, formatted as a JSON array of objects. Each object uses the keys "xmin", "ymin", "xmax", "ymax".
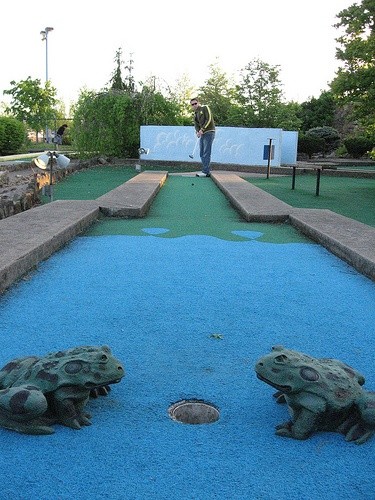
[{"xmin": 279, "ymin": 162, "xmax": 336, "ymax": 198}]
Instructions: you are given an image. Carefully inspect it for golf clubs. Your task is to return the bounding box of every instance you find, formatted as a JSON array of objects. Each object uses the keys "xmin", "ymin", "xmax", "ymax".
[{"xmin": 189, "ymin": 135, "xmax": 201, "ymax": 158}]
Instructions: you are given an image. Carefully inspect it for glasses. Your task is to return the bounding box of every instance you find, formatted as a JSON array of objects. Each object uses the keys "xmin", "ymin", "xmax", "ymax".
[{"xmin": 192, "ymin": 103, "xmax": 197, "ymax": 105}]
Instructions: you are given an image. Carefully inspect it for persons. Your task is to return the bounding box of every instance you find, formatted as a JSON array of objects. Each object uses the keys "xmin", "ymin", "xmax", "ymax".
[
  {"xmin": 53, "ymin": 124, "xmax": 68, "ymax": 145},
  {"xmin": 190, "ymin": 98, "xmax": 216, "ymax": 178}
]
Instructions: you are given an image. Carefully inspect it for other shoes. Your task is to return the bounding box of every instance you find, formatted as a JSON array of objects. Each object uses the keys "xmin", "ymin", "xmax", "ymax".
[{"xmin": 196, "ymin": 172, "xmax": 210, "ymax": 177}]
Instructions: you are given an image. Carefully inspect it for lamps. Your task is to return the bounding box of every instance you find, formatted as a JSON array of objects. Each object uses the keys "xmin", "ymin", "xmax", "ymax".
[
  {"xmin": 136, "ymin": 147, "xmax": 149, "ymax": 174},
  {"xmin": 34, "ymin": 151, "xmax": 71, "ymax": 202}
]
